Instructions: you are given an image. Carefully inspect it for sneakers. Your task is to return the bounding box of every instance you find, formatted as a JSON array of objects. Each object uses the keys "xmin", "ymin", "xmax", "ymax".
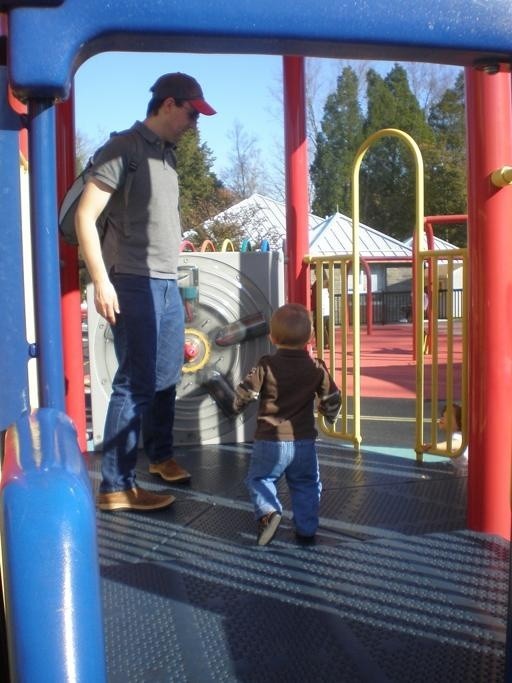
[
  {"xmin": 294, "ymin": 533, "xmax": 318, "ymax": 544},
  {"xmin": 96, "ymin": 487, "xmax": 176, "ymax": 511},
  {"xmin": 148, "ymin": 457, "xmax": 193, "ymax": 485},
  {"xmin": 257, "ymin": 509, "xmax": 283, "ymax": 547}
]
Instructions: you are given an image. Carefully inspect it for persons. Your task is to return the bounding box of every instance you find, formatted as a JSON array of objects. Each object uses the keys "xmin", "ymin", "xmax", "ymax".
[
  {"xmin": 399, "ymin": 289, "xmax": 413, "ymax": 322},
  {"xmin": 68, "ymin": 71, "xmax": 219, "ymax": 511},
  {"xmin": 234, "ymin": 304, "xmax": 344, "ymax": 547},
  {"xmin": 414, "ymin": 402, "xmax": 467, "ymax": 466}
]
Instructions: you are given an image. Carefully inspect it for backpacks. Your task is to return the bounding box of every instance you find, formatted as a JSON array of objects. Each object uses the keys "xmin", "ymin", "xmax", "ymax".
[{"xmin": 59, "ymin": 129, "xmax": 145, "ymax": 247}]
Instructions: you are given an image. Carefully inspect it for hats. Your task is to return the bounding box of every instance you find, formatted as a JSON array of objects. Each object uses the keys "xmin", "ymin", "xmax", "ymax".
[{"xmin": 151, "ymin": 72, "xmax": 216, "ymax": 115}]
